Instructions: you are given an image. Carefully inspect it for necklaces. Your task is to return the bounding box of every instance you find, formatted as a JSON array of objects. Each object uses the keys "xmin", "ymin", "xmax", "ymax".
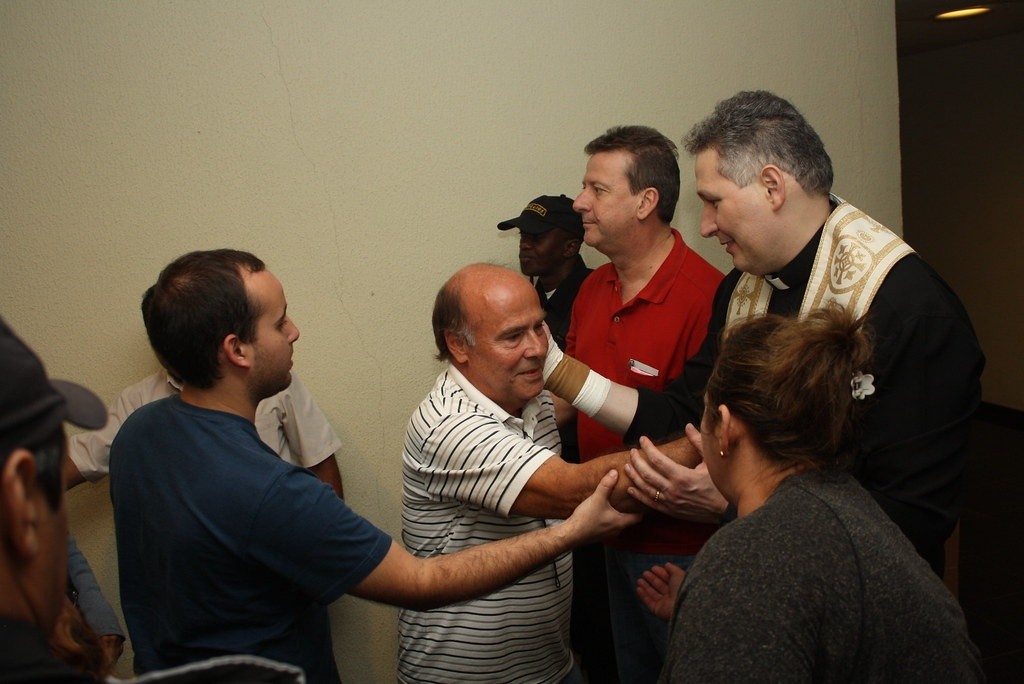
[{"xmin": 522, "ymin": 431, "xmax": 561, "ymax": 588}]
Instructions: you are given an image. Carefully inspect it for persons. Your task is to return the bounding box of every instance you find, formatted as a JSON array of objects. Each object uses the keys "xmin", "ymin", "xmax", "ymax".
[
  {"xmin": 108, "ymin": 248, "xmax": 643, "ymax": 684},
  {"xmin": 0, "ymin": 319, "xmax": 127, "ymax": 684},
  {"xmin": 552, "ymin": 126, "xmax": 730, "ymax": 684},
  {"xmin": 543, "ymin": 90, "xmax": 986, "ymax": 649},
  {"xmin": 497, "ymin": 194, "xmax": 597, "ymax": 462},
  {"xmin": 394, "ymin": 262, "xmax": 702, "ymax": 684},
  {"xmin": 64, "ymin": 365, "xmax": 346, "ymax": 504},
  {"xmin": 634, "ymin": 307, "xmax": 987, "ymax": 684}
]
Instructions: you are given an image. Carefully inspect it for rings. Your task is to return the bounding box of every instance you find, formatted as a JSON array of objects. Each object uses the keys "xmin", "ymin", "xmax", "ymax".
[{"xmin": 654, "ymin": 490, "xmax": 659, "ymax": 502}]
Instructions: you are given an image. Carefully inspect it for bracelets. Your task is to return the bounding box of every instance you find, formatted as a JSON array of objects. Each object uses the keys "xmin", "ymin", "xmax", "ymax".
[{"xmin": 718, "ymin": 504, "xmax": 737, "ymax": 528}]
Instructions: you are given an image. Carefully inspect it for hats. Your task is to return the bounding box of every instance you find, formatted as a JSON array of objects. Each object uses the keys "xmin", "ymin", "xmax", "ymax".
[
  {"xmin": 0, "ymin": 320, "xmax": 107, "ymax": 464},
  {"xmin": 497, "ymin": 192, "xmax": 584, "ymax": 240}
]
